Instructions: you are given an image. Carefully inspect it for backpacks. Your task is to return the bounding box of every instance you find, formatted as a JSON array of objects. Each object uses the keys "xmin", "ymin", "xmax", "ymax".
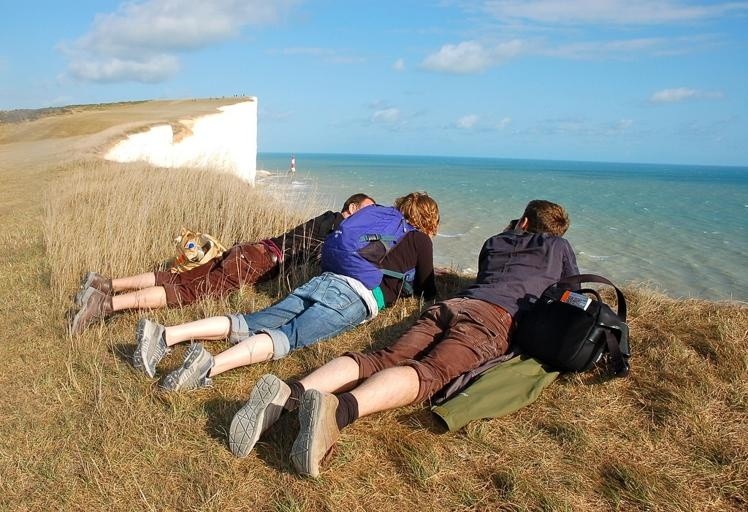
[
  {"xmin": 172, "ymin": 228, "xmax": 227, "ymax": 274},
  {"xmin": 516, "ymin": 274, "xmax": 632, "ymax": 378},
  {"xmin": 321, "ymin": 204, "xmax": 418, "ymax": 290}
]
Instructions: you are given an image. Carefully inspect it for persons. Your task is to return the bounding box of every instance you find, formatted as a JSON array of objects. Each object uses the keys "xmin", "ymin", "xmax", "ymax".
[
  {"xmin": 128, "ymin": 185, "xmax": 444, "ymax": 396},
  {"xmin": 227, "ymin": 197, "xmax": 584, "ymax": 480},
  {"xmin": 64, "ymin": 191, "xmax": 377, "ymax": 345}
]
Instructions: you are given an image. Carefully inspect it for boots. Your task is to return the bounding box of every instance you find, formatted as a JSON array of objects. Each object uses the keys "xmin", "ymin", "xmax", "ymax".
[{"xmin": 71, "ymin": 272, "xmax": 114, "ymax": 336}]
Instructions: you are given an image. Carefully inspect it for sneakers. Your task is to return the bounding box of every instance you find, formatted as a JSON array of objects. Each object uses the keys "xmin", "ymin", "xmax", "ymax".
[
  {"xmin": 133, "ymin": 319, "xmax": 167, "ymax": 379},
  {"xmin": 289, "ymin": 389, "xmax": 342, "ymax": 478},
  {"xmin": 163, "ymin": 343, "xmax": 214, "ymax": 392},
  {"xmin": 229, "ymin": 374, "xmax": 292, "ymax": 458}
]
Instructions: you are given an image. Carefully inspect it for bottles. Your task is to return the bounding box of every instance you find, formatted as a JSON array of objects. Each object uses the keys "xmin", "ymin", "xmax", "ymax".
[{"xmin": 189, "ymin": 243, "xmax": 204, "ymax": 259}]
